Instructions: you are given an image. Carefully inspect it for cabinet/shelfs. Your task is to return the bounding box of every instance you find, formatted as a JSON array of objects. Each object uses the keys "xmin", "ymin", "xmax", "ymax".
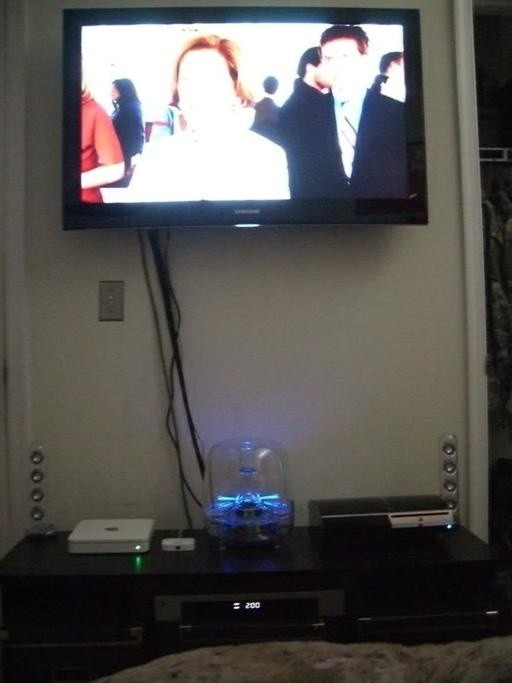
[{"xmin": 1, "ymin": 524, "xmax": 510, "ymax": 669}]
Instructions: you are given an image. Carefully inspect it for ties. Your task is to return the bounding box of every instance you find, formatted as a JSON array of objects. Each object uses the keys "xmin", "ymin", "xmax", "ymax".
[{"xmin": 338, "ymin": 100, "xmax": 359, "ymax": 179}]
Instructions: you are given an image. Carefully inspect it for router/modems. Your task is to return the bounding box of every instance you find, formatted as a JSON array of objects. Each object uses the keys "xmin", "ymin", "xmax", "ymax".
[{"xmin": 68, "ymin": 517, "xmax": 154, "ymax": 552}]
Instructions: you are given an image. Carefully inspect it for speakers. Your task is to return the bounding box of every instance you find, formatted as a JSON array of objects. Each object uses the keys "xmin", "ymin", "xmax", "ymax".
[
  {"xmin": 436, "ymin": 432, "xmax": 460, "ymax": 528},
  {"xmin": 27, "ymin": 440, "xmax": 47, "ymax": 535}
]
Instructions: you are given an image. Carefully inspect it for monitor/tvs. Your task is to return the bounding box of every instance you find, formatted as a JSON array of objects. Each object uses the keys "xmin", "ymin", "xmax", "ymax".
[{"xmin": 59, "ymin": 4, "xmax": 431, "ymax": 232}]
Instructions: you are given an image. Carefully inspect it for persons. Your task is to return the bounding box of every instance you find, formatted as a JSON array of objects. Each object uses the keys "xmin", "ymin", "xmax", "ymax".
[
  {"xmin": 104, "ymin": 77, "xmax": 148, "ymax": 184},
  {"xmin": 249, "ymin": 73, "xmax": 284, "ymax": 138},
  {"xmin": 110, "ymin": 33, "xmax": 294, "ymax": 201},
  {"xmin": 272, "ymin": 43, "xmax": 327, "ymax": 122},
  {"xmin": 76, "ymin": 81, "xmax": 126, "ymax": 204},
  {"xmin": 281, "ymin": 24, "xmax": 413, "ymax": 201},
  {"xmin": 375, "ymin": 49, "xmax": 407, "ymax": 103}
]
